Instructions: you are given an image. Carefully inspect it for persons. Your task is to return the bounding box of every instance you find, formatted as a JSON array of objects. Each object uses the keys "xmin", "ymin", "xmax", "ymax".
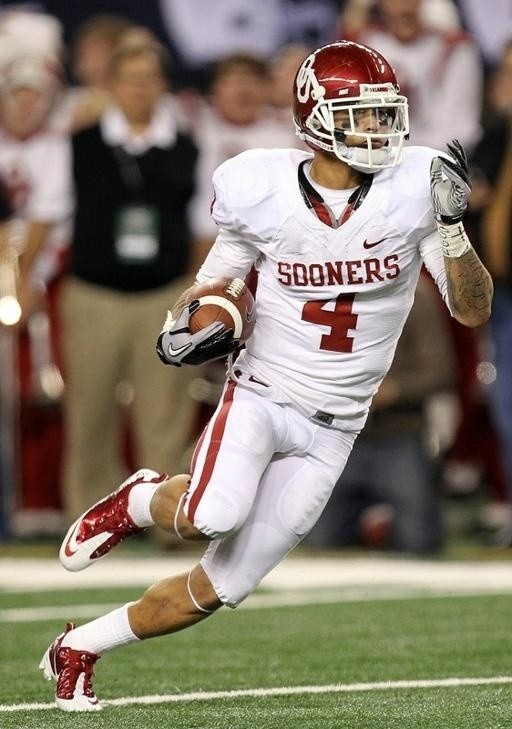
[
  {"xmin": 33, "ymin": 35, "xmax": 498, "ymax": 710},
  {"xmin": 1, "ymin": 0, "xmax": 512, "ymax": 561}
]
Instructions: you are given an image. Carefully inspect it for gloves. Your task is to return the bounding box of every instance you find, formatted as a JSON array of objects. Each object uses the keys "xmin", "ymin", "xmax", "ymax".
[
  {"xmin": 157, "ymin": 300, "xmax": 245, "ymax": 367},
  {"xmin": 430, "ymin": 140, "xmax": 472, "ymax": 224}
]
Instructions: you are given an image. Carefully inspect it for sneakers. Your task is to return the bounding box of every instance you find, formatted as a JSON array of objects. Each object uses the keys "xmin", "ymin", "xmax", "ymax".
[
  {"xmin": 39, "ymin": 622, "xmax": 104, "ymax": 712},
  {"xmin": 59, "ymin": 469, "xmax": 168, "ymax": 571}
]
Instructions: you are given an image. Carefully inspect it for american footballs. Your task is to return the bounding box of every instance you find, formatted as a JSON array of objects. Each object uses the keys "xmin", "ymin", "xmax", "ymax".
[{"xmin": 190, "ymin": 277, "xmax": 256, "ymax": 342}]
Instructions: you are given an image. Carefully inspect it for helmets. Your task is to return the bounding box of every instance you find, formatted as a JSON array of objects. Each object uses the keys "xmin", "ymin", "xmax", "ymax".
[{"xmin": 294, "ymin": 42, "xmax": 410, "ymax": 174}]
